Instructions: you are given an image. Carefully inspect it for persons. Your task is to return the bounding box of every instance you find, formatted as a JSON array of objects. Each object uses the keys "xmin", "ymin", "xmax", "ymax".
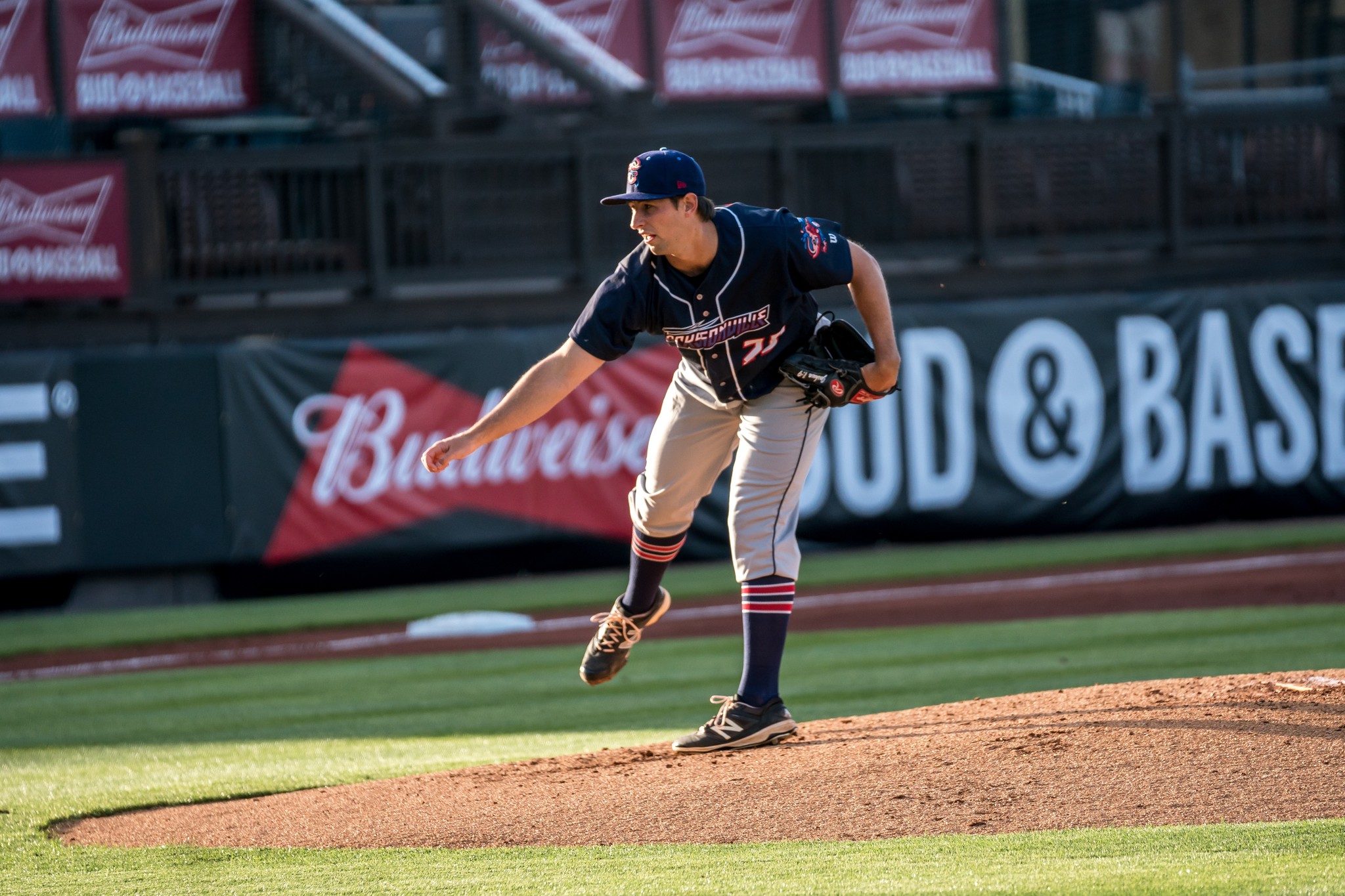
[{"xmin": 420, "ymin": 145, "xmax": 900, "ymax": 753}]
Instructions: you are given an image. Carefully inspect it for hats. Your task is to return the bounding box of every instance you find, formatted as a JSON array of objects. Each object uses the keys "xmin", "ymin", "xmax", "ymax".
[{"xmin": 600, "ymin": 146, "xmax": 707, "ymax": 207}]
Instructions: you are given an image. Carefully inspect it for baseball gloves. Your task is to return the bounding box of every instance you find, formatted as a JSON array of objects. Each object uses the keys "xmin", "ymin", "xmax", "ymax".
[{"xmin": 779, "ymin": 319, "xmax": 898, "ymax": 409}]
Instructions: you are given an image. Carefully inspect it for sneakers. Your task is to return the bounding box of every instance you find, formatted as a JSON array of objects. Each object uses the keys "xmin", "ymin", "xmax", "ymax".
[
  {"xmin": 671, "ymin": 692, "xmax": 797, "ymax": 753},
  {"xmin": 580, "ymin": 585, "xmax": 672, "ymax": 686}
]
